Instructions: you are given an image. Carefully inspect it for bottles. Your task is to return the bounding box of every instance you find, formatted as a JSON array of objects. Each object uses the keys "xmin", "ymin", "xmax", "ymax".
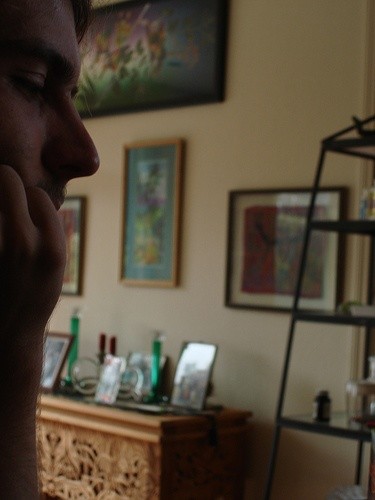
[{"xmin": 313, "ymin": 390, "xmax": 331, "ymax": 422}]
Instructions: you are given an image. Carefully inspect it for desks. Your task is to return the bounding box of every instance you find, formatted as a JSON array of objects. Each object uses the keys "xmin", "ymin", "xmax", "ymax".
[{"xmin": 34, "ymin": 391, "xmax": 255, "ymax": 500}]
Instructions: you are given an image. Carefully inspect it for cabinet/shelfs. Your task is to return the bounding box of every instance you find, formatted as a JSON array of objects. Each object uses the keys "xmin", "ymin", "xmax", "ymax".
[{"xmin": 264, "ymin": 115, "xmax": 375, "ymax": 500}]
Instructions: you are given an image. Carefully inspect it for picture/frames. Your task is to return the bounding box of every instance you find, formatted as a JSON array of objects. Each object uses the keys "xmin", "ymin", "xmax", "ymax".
[
  {"xmin": 224, "ymin": 186, "xmax": 346, "ymax": 317},
  {"xmin": 94, "ymin": 354, "xmax": 128, "ymax": 406},
  {"xmin": 39, "ymin": 331, "xmax": 76, "ymax": 392},
  {"xmin": 168, "ymin": 339, "xmax": 218, "ymax": 412},
  {"xmin": 57, "ymin": 194, "xmax": 86, "ymax": 298},
  {"xmin": 72, "ymin": 0, "xmax": 228, "ymax": 119},
  {"xmin": 119, "ymin": 136, "xmax": 185, "ymax": 287}
]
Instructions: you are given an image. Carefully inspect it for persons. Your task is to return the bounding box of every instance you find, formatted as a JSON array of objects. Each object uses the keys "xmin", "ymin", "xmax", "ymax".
[{"xmin": 0, "ymin": 0, "xmax": 100, "ymax": 500}]
